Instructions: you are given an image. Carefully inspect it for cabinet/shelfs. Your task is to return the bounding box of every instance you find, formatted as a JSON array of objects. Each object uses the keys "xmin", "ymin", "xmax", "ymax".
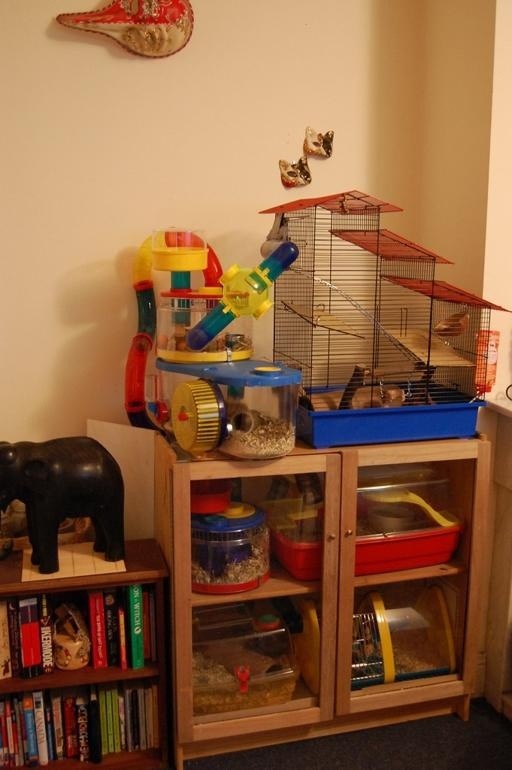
[
  {"xmin": 0, "ymin": 542, "xmax": 170, "ymax": 770},
  {"xmin": 485, "ymin": 398, "xmax": 512, "ymax": 728},
  {"xmin": 156, "ymin": 434, "xmax": 490, "ymax": 770}
]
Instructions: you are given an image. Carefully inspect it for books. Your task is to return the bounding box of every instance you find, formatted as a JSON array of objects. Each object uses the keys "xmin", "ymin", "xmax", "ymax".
[
  {"xmin": 0, "ymin": 676, "xmax": 160, "ymax": 765},
  {"xmin": 1, "ymin": 582, "xmax": 158, "ymax": 680}
]
